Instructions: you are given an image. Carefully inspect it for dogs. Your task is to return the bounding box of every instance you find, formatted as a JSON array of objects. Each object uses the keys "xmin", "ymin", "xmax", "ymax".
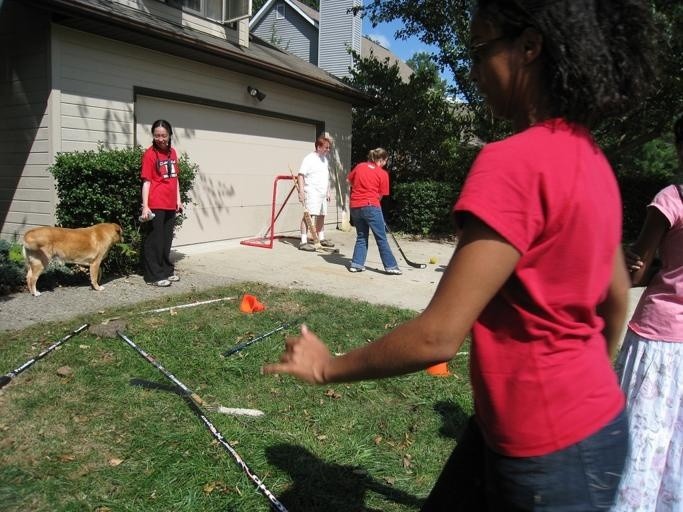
[{"xmin": 22, "ymin": 222, "xmax": 126, "ymax": 297}]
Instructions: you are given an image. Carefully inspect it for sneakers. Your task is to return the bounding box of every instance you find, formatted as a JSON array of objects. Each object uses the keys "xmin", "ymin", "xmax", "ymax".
[
  {"xmin": 169, "ymin": 276, "xmax": 179, "ymax": 281},
  {"xmin": 350, "ymin": 267, "xmax": 365, "ymax": 272},
  {"xmin": 147, "ymin": 280, "xmax": 171, "ymax": 287},
  {"xmin": 320, "ymin": 240, "xmax": 334, "ymax": 247},
  {"xmin": 386, "ymin": 268, "xmax": 402, "ymax": 274},
  {"xmin": 299, "ymin": 243, "xmax": 316, "ymax": 252}
]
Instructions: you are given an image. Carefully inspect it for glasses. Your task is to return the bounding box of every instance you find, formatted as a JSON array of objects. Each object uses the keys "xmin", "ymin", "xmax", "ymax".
[{"xmin": 468, "ymin": 34, "xmax": 505, "ymax": 61}]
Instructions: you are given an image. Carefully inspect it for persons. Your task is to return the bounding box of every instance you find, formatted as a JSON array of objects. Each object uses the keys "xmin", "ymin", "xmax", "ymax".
[
  {"xmin": 344, "ymin": 145, "xmax": 403, "ymax": 276},
  {"xmin": 610, "ymin": 112, "xmax": 682, "ymax": 511},
  {"xmin": 139, "ymin": 117, "xmax": 184, "ymax": 289},
  {"xmin": 258, "ymin": 0, "xmax": 634, "ymax": 510},
  {"xmin": 295, "ymin": 135, "xmax": 336, "ymax": 252}
]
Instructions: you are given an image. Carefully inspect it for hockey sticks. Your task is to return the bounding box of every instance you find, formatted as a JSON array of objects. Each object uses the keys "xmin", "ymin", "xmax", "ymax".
[
  {"xmin": 101, "ymin": 296, "xmax": 236, "ymax": 325},
  {"xmin": 287, "ymin": 162, "xmax": 339, "ymax": 253},
  {"xmin": 384, "ymin": 220, "xmax": 427, "ymax": 269},
  {"xmin": 116, "ymin": 330, "xmax": 264, "ymax": 416}
]
